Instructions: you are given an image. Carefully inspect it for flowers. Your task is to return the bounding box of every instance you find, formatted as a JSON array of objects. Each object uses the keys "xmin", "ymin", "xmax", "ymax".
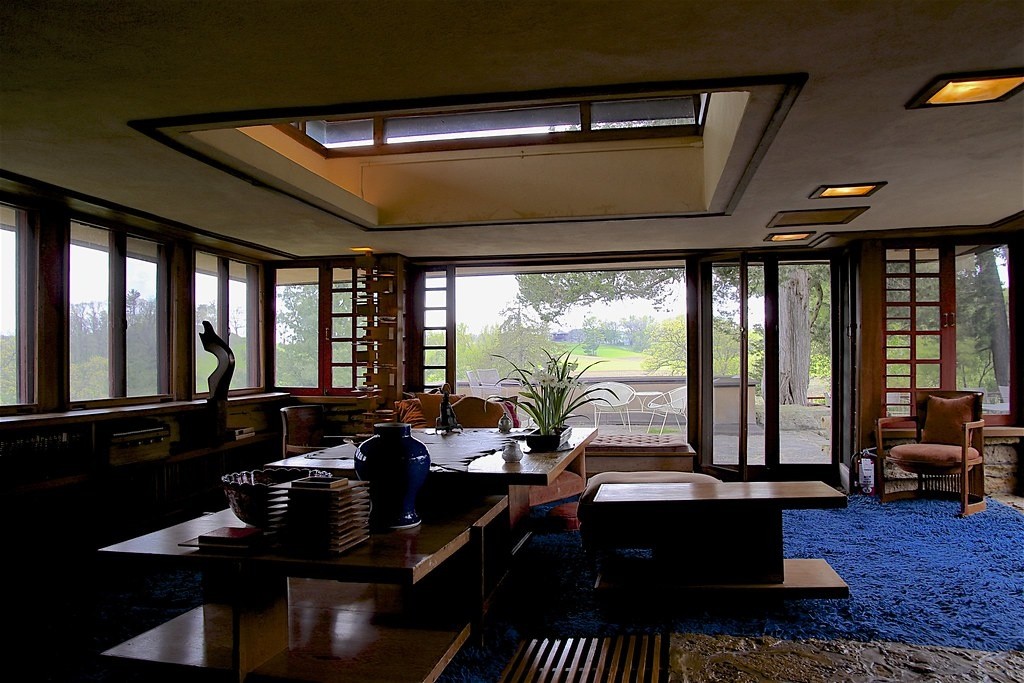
[{"xmin": 491, "ymin": 348, "xmax": 620, "ymax": 435}]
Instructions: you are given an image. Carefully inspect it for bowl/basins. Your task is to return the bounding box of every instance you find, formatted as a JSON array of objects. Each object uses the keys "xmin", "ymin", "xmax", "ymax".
[{"xmin": 221, "ymin": 467, "xmax": 333, "ymax": 531}]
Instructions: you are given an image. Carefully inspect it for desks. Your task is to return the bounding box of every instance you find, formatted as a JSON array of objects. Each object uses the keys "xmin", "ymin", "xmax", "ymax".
[
  {"xmin": 264, "ymin": 428, "xmax": 599, "ymax": 554},
  {"xmin": 99, "ymin": 492, "xmax": 506, "ymax": 683},
  {"xmin": 593, "ymin": 479, "xmax": 851, "ymax": 597},
  {"xmin": 634, "ymin": 391, "xmax": 663, "ymax": 411}
]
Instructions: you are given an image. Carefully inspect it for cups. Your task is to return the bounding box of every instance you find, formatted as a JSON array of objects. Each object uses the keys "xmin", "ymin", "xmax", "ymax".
[{"xmin": 355, "ymin": 385, "xmax": 374, "ymax": 391}]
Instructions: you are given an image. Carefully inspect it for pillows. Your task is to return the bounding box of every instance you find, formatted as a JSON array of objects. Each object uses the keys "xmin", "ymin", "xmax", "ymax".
[{"xmin": 417, "ymin": 392, "xmax": 465, "ymax": 428}]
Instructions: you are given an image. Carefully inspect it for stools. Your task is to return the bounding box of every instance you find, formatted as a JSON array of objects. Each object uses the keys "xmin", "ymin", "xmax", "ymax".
[{"xmin": 575, "ymin": 470, "xmax": 722, "ymax": 550}]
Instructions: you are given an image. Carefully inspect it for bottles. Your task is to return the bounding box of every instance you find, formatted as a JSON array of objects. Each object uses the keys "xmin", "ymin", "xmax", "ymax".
[
  {"xmin": 354, "ymin": 423, "xmax": 431, "ymax": 531},
  {"xmin": 502, "ymin": 442, "xmax": 523, "ymax": 463},
  {"xmin": 498, "ymin": 412, "xmax": 512, "ymax": 433}
]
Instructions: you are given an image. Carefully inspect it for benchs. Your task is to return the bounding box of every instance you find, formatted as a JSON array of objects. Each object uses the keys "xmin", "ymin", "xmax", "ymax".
[{"xmin": 583, "ymin": 435, "xmax": 696, "ymax": 479}]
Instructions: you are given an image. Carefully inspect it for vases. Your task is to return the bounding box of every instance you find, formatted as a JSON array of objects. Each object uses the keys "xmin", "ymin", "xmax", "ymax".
[
  {"xmin": 354, "ymin": 423, "xmax": 431, "ymax": 531},
  {"xmin": 524, "ymin": 424, "xmax": 572, "ymax": 451}
]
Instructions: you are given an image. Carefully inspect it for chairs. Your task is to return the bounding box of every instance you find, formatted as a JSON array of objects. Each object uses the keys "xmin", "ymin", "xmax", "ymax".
[
  {"xmin": 874, "ymin": 390, "xmax": 985, "ymax": 516},
  {"xmin": 581, "ymin": 382, "xmax": 688, "ymax": 435},
  {"xmin": 279, "ymin": 405, "xmax": 355, "ymax": 460},
  {"xmin": 464, "ymin": 368, "xmax": 508, "ymax": 402}
]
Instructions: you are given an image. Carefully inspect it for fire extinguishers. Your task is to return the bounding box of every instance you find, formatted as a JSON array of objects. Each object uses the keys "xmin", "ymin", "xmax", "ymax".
[{"xmin": 850, "ymin": 447, "xmax": 878, "ymax": 497}]
